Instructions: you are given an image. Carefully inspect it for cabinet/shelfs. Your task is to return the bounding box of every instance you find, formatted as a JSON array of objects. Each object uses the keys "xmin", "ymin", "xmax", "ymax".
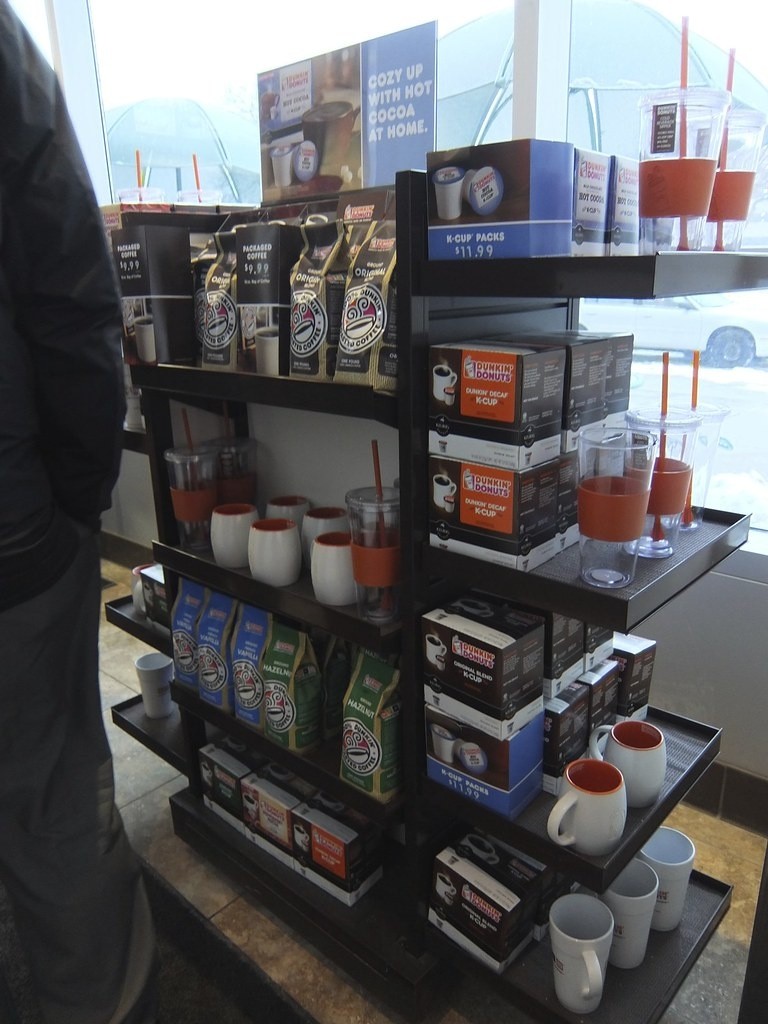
[{"xmin": 106, "ymin": 171, "xmax": 768, "ymax": 1024}]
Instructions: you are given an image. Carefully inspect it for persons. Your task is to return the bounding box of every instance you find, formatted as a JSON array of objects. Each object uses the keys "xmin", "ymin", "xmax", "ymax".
[{"xmin": 0, "ymin": 1, "xmax": 159, "ymax": 1024}]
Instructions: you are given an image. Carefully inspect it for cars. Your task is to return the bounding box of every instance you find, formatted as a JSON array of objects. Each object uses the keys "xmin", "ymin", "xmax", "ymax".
[{"xmin": 578, "ymin": 287, "xmax": 768, "ymax": 370}]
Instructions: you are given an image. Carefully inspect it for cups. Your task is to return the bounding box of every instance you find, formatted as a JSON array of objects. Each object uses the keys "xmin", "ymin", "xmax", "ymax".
[
  {"xmin": 460, "ymin": 834, "xmax": 499, "ymax": 873},
  {"xmin": 133, "ymin": 316, "xmax": 156, "ymax": 363},
  {"xmin": 624, "ymin": 407, "xmax": 704, "ymax": 559},
  {"xmin": 293, "ymin": 824, "xmax": 310, "ymax": 847},
  {"xmin": 589, "ymin": 721, "xmax": 666, "ymax": 807},
  {"xmin": 433, "ymin": 166, "xmax": 467, "ymax": 219},
  {"xmin": 177, "ymin": 189, "xmax": 222, "ymax": 205},
  {"xmin": 254, "ymin": 327, "xmax": 279, "ymax": 377},
  {"xmin": 604, "ymin": 857, "xmax": 659, "ymax": 969},
  {"xmin": 425, "ymin": 633, "xmax": 449, "ymax": 667},
  {"xmin": 247, "ymin": 519, "xmax": 302, "ymax": 586},
  {"xmin": 462, "ymin": 165, "xmax": 504, "ymax": 216},
  {"xmin": 266, "ymin": 496, "xmax": 312, "ymax": 537},
  {"xmin": 200, "ymin": 763, "xmax": 214, "ymax": 786},
  {"xmin": 293, "ymin": 140, "xmax": 318, "ymax": 183},
  {"xmin": 211, "ymin": 504, "xmax": 259, "ymax": 568},
  {"xmin": 433, "ymin": 473, "xmax": 457, "ymax": 508},
  {"xmin": 269, "ymin": 146, "xmax": 294, "ymax": 187},
  {"xmin": 641, "ymin": 826, "xmax": 696, "ymax": 930},
  {"xmin": 547, "ymin": 758, "xmax": 627, "ymax": 855},
  {"xmin": 702, "ymin": 106, "xmax": 768, "ymax": 254},
  {"xmin": 346, "ymin": 486, "xmax": 400, "ymax": 623},
  {"xmin": 431, "ymin": 723, "xmax": 456, "ymax": 764},
  {"xmin": 578, "ymin": 427, "xmax": 659, "ymax": 589},
  {"xmin": 242, "ymin": 793, "xmax": 259, "ymax": 819},
  {"xmin": 549, "ymin": 894, "xmax": 614, "ymax": 1015},
  {"xmin": 303, "ymin": 507, "xmax": 350, "ymax": 539},
  {"xmin": 218, "ymin": 435, "xmax": 258, "ymax": 504},
  {"xmin": 311, "ymin": 530, "xmax": 358, "ymax": 605},
  {"xmin": 435, "ymin": 872, "xmax": 457, "ymax": 906},
  {"xmin": 118, "ymin": 187, "xmax": 165, "ymax": 202},
  {"xmin": 301, "ymin": 101, "xmax": 361, "ymax": 175},
  {"xmin": 132, "ymin": 564, "xmax": 154, "ymax": 616},
  {"xmin": 638, "ymin": 86, "xmax": 732, "ymax": 254},
  {"xmin": 432, "ymin": 364, "xmax": 458, "ymax": 401},
  {"xmin": 135, "ymin": 652, "xmax": 174, "ymax": 719},
  {"xmin": 455, "ymin": 738, "xmax": 488, "ymax": 774},
  {"xmin": 165, "ymin": 444, "xmax": 221, "ymax": 554},
  {"xmin": 679, "ymin": 401, "xmax": 733, "ymax": 532}
]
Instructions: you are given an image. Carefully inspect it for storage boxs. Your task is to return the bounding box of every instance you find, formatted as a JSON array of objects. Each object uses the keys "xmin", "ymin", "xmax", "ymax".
[
  {"xmin": 427, "ymin": 330, "xmax": 634, "ymax": 572},
  {"xmin": 97, "ymin": 203, "xmax": 257, "ymax": 365},
  {"xmin": 604, "ymin": 156, "xmax": 640, "ymax": 257},
  {"xmin": 198, "ymin": 736, "xmax": 384, "ymax": 907},
  {"xmin": 422, "ymin": 588, "xmax": 657, "ymax": 820},
  {"xmin": 426, "ymin": 139, "xmax": 575, "ymax": 259},
  {"xmin": 428, "ymin": 825, "xmax": 581, "ymax": 975},
  {"xmin": 571, "ymin": 147, "xmax": 611, "ymax": 258}
]
